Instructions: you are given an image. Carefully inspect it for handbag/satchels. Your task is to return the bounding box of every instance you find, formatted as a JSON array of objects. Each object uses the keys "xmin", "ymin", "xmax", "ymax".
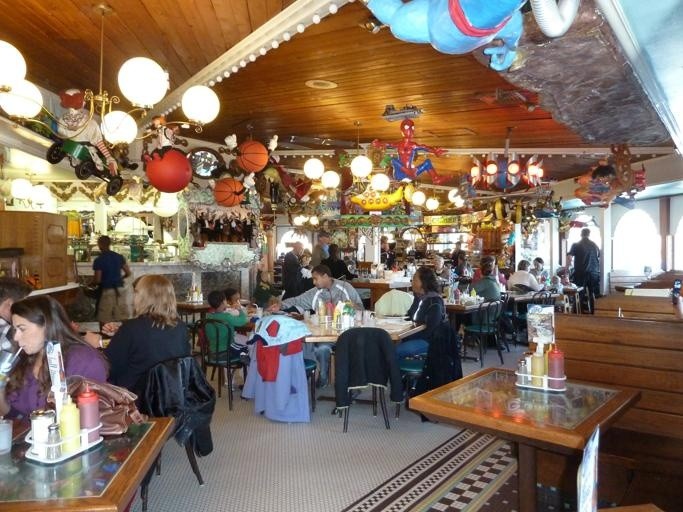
[{"xmin": 46, "ymin": 374, "xmax": 149, "ymax": 436}]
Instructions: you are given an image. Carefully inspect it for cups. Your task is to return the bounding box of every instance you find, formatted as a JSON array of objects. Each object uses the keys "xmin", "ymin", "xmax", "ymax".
[
  {"xmin": 355, "ymin": 308, "xmax": 362, "ymax": 321},
  {"xmin": 0, "ymin": 420, "xmax": 13, "ymax": 455},
  {"xmin": 0, "ymin": 351, "xmax": 20, "ymax": 390},
  {"xmin": 255, "ymin": 307, "xmax": 263, "ymax": 317},
  {"xmin": 304, "ymin": 308, "xmax": 316, "ymax": 320},
  {"xmin": 354, "ymin": 264, "xmax": 384, "ymax": 279},
  {"xmin": 363, "ymin": 310, "xmax": 370, "ymax": 321}
]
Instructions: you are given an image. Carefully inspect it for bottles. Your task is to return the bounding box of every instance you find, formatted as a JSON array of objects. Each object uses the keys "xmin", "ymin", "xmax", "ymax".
[
  {"xmin": 443, "ymin": 286, "xmax": 477, "ymax": 303},
  {"xmin": 29, "ymin": 382, "xmax": 100, "ymax": 460},
  {"xmin": 11, "ymin": 261, "xmax": 33, "ymax": 279},
  {"xmin": 158, "ymin": 250, "xmax": 166, "ymax": 262},
  {"xmin": 537, "ymin": 275, "xmax": 551, "ymax": 285},
  {"xmin": 644, "ymin": 266, "xmax": 652, "ymax": 277},
  {"xmin": 516, "ymin": 343, "xmax": 565, "ymax": 389},
  {"xmin": 161, "ymin": 247, "xmax": 170, "ymax": 261},
  {"xmin": 185, "ymin": 282, "xmax": 203, "ymax": 302},
  {"xmin": 319, "ymin": 300, "xmax": 355, "ymax": 330},
  {"xmin": 459, "ymin": 235, "xmax": 464, "ymax": 242}
]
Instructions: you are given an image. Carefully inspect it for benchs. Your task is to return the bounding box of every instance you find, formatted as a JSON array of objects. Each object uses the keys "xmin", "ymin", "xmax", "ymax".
[{"xmin": 528, "ymin": 271, "xmax": 683, "ymax": 512}]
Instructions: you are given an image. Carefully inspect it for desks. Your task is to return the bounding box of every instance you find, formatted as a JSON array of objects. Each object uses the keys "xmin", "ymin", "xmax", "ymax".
[
  {"xmin": 0, "ymin": 416, "xmax": 175, "ymax": 512},
  {"xmin": 408, "ymin": 368, "xmax": 642, "ymax": 512}
]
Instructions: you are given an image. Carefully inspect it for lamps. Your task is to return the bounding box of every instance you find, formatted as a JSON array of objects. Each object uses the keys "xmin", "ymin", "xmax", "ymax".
[
  {"xmin": 0, "ymin": 0, "xmax": 221, "ymax": 156},
  {"xmin": 10, "ymin": 172, "xmax": 51, "ymax": 205},
  {"xmin": 411, "ymin": 176, "xmax": 464, "ymax": 213},
  {"xmin": 293, "ymin": 204, "xmax": 321, "ymax": 229},
  {"xmin": 303, "ymin": 121, "xmax": 390, "ymax": 195},
  {"xmin": 153, "ymin": 191, "xmax": 179, "ymax": 219},
  {"xmin": 470, "ymin": 148, "xmax": 547, "ymax": 187}
]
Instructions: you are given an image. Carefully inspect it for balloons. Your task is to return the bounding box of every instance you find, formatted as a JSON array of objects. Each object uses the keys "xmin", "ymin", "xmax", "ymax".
[{"xmin": 371, "ymin": 119, "xmax": 454, "ymax": 186}]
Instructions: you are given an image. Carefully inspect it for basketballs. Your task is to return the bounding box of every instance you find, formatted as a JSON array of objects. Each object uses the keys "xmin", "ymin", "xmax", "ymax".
[
  {"xmin": 215, "ymin": 179, "xmax": 244, "ymax": 207},
  {"xmin": 237, "ymin": 141, "xmax": 268, "ymax": 173}
]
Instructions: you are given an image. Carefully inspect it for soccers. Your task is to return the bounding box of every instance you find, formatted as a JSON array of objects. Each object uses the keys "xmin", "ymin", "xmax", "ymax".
[{"xmin": 145, "ymin": 149, "xmax": 191, "ymax": 192}]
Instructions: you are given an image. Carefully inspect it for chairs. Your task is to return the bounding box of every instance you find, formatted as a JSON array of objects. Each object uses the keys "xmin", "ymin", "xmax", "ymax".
[{"xmin": 131, "ymin": 356, "xmax": 203, "ymax": 512}]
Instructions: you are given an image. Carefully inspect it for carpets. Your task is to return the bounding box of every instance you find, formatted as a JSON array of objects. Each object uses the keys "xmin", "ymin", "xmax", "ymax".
[{"xmin": 337, "ymin": 427, "xmax": 621, "ymax": 512}]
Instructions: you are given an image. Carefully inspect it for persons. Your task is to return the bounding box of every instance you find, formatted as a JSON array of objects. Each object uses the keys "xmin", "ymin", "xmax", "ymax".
[
  {"xmin": 449, "ymin": 262, "xmax": 501, "ymax": 331},
  {"xmin": 83, "ymin": 274, "xmax": 190, "ymax": 412},
  {"xmin": 393, "ymin": 266, "xmax": 448, "ymax": 358},
  {"xmin": 568, "ymin": 228, "xmax": 601, "ymax": 316},
  {"xmin": 278, "ymin": 233, "xmax": 472, "ymax": 299},
  {"xmin": 0, "ymin": 276, "xmax": 33, "ymax": 352},
  {"xmin": 88, "ymin": 234, "xmax": 132, "ymax": 329},
  {"xmin": 506, "ymin": 253, "xmax": 579, "ymax": 312},
  {"xmin": 247, "ymin": 264, "xmax": 364, "ymax": 390},
  {"xmin": 472, "ymin": 256, "xmax": 507, "ymax": 290},
  {"xmin": 204, "ymin": 289, "xmax": 250, "ymax": 391},
  {"xmin": 0, "ymin": 294, "xmax": 110, "ymax": 421}
]
